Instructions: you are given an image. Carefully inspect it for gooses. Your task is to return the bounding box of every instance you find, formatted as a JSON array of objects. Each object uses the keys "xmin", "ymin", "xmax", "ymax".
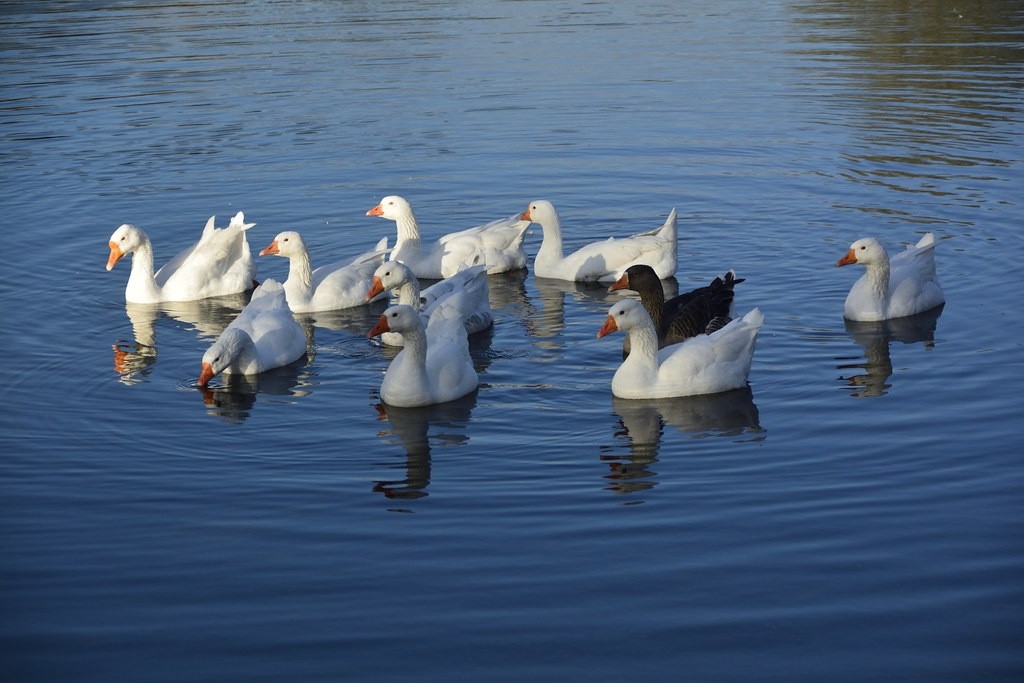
[
  {"xmin": 258, "ymin": 230, "xmax": 396, "ymax": 314},
  {"xmin": 105, "ymin": 210, "xmax": 257, "ymax": 304},
  {"xmin": 607, "ymin": 265, "xmax": 746, "ymax": 352},
  {"xmin": 366, "ymin": 304, "xmax": 479, "ymax": 408},
  {"xmin": 597, "ymin": 299, "xmax": 765, "ymax": 400},
  {"xmin": 201, "ymin": 353, "xmax": 320, "ymax": 410},
  {"xmin": 363, "ymin": 261, "xmax": 493, "ymax": 347},
  {"xmin": 365, "ymin": 195, "xmax": 534, "ymax": 279},
  {"xmin": 520, "ymin": 199, "xmax": 679, "ymax": 282},
  {"xmin": 599, "ymin": 386, "xmax": 768, "ymax": 482},
  {"xmin": 837, "ymin": 303, "xmax": 945, "ymax": 384},
  {"xmin": 198, "ymin": 277, "xmax": 305, "ymax": 388},
  {"xmin": 835, "ymin": 233, "xmax": 945, "ymax": 321},
  {"xmin": 374, "ymin": 385, "xmax": 483, "ymax": 498},
  {"xmin": 110, "ymin": 282, "xmax": 261, "ymax": 375}
]
